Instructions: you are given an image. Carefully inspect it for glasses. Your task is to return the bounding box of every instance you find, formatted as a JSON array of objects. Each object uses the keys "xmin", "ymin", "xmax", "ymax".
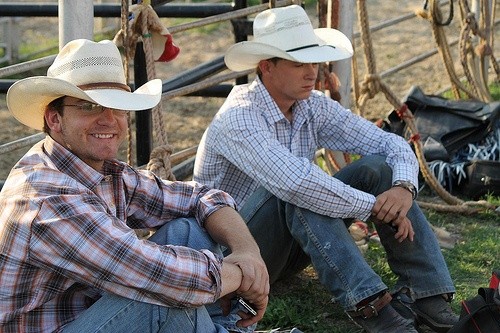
[{"xmin": 59, "ymin": 101, "xmax": 130, "ymax": 114}]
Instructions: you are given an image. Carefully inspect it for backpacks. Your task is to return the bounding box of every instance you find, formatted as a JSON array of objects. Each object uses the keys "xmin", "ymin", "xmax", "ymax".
[
  {"xmin": 445, "ymin": 286, "xmax": 500, "ymax": 333},
  {"xmin": 379, "ymin": 86, "xmax": 500, "ymax": 198}
]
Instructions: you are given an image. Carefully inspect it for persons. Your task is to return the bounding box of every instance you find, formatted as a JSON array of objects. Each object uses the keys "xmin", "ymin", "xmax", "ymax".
[
  {"xmin": 0, "ymin": 39, "xmax": 270, "ymax": 333},
  {"xmin": 192, "ymin": 4, "xmax": 461, "ymax": 333}
]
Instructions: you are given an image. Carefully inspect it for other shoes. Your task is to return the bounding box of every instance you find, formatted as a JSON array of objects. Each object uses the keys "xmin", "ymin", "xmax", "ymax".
[
  {"xmin": 345, "ymin": 291, "xmax": 416, "ymax": 333},
  {"xmin": 398, "ymin": 289, "xmax": 460, "ymax": 328}
]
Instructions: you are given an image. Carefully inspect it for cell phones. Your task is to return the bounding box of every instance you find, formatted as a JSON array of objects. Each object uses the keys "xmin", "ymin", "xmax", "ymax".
[
  {"xmin": 382, "ymin": 222, "xmax": 398, "ymax": 234},
  {"xmin": 231, "ymin": 297, "xmax": 257, "ymax": 317}
]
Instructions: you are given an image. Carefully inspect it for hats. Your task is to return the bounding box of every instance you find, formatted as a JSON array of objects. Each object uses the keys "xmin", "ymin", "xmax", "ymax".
[
  {"xmin": 5, "ymin": 38, "xmax": 162, "ymax": 132},
  {"xmin": 224, "ymin": 4, "xmax": 354, "ymax": 72}
]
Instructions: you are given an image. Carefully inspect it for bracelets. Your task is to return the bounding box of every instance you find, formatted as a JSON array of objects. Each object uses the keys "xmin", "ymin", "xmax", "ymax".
[{"xmin": 393, "ymin": 181, "xmax": 417, "ymax": 200}]
